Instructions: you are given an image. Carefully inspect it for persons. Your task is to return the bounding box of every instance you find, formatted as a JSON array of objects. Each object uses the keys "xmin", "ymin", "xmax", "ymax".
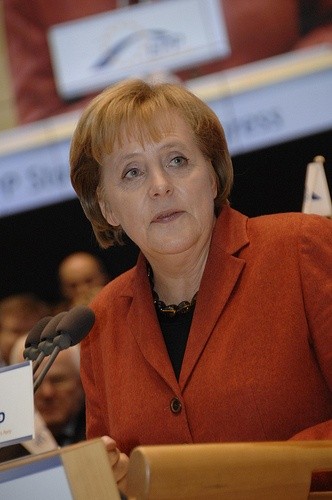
[
  {"xmin": 0, "ymin": 252, "xmax": 103, "ymax": 464},
  {"xmin": 69, "ymin": 77, "xmax": 332, "ymax": 500}
]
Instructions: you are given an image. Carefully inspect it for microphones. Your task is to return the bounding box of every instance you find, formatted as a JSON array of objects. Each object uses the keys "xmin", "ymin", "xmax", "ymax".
[
  {"xmin": 33, "ymin": 311, "xmax": 69, "ymax": 377},
  {"xmin": 23, "ymin": 317, "xmax": 53, "ymax": 362},
  {"xmin": 34, "ymin": 306, "xmax": 95, "ymax": 393}
]
